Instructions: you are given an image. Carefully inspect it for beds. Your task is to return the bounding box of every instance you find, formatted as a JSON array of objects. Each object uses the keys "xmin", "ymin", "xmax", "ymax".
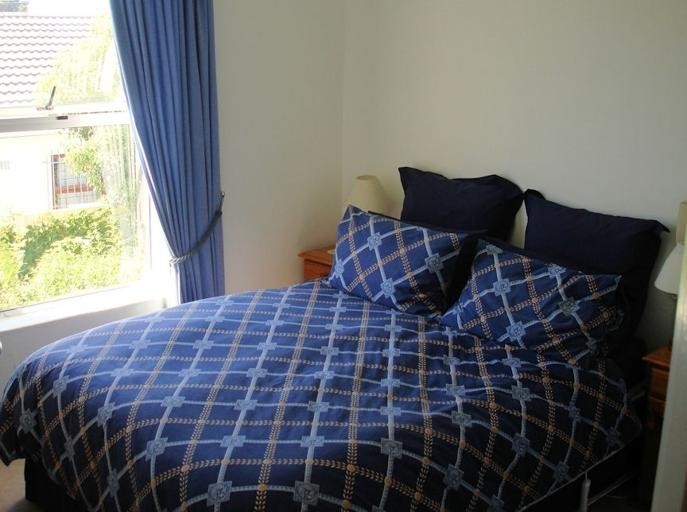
[{"xmin": 0, "ymin": 233, "xmax": 678, "ymax": 512}]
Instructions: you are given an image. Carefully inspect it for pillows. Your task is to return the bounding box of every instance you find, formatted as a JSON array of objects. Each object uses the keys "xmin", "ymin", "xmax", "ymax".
[
  {"xmin": 439, "ymin": 237, "xmax": 622, "ymax": 374},
  {"xmin": 327, "ymin": 205, "xmax": 486, "ymax": 321},
  {"xmin": 398, "ymin": 166, "xmax": 525, "ymax": 247},
  {"xmin": 520, "ymin": 189, "xmax": 671, "ymax": 355}
]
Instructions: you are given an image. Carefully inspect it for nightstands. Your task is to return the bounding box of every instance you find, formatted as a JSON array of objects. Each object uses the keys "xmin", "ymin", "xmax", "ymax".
[
  {"xmin": 297, "ymin": 244, "xmax": 336, "ymax": 281},
  {"xmin": 641, "ymin": 342, "xmax": 670, "ymax": 419}
]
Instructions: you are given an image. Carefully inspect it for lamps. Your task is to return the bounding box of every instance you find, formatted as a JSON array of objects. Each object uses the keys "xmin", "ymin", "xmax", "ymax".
[{"xmin": 654, "ymin": 242, "xmax": 681, "ymax": 351}]
